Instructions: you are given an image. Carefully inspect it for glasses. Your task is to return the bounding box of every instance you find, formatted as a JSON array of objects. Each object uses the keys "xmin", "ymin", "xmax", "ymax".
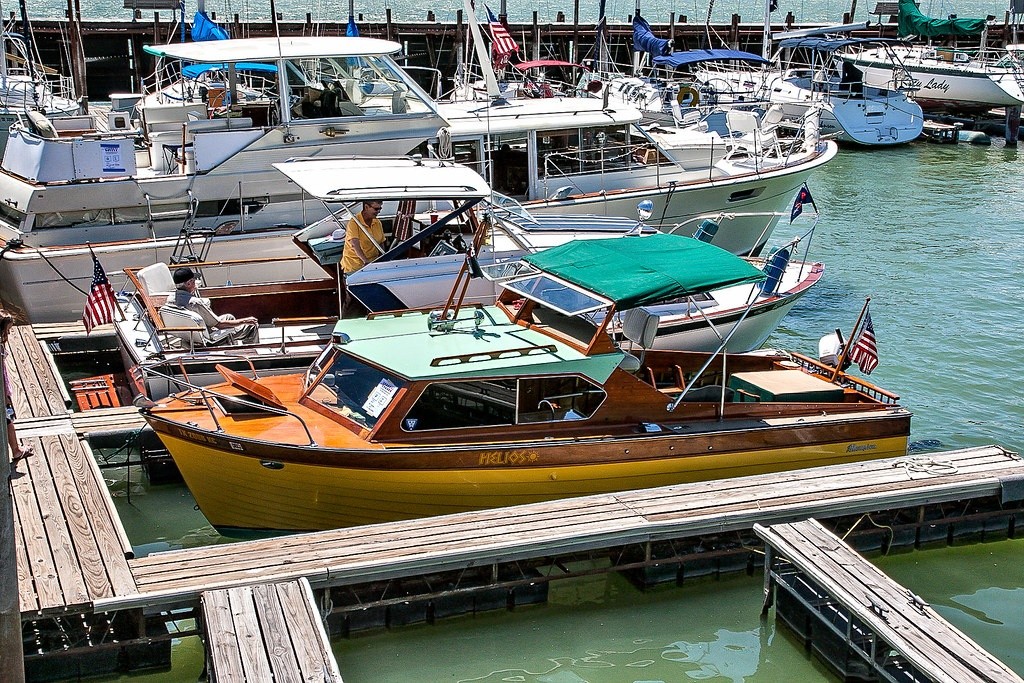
[{"xmin": 367, "ymin": 204, "xmax": 382, "ymax": 211}]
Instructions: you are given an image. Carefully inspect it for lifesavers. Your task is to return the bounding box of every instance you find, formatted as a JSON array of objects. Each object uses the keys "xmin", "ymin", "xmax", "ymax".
[{"xmin": 676, "ymin": 87, "xmax": 699, "ymax": 107}]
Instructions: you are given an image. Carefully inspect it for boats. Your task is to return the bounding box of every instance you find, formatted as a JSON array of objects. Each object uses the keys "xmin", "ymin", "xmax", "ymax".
[
  {"xmin": 0, "ymin": 22, "xmax": 82, "ymax": 161},
  {"xmin": 0, "ymin": 1, "xmax": 838, "ymax": 326},
  {"xmin": 132, "ymin": 235, "xmax": 913, "ymax": 540},
  {"xmin": 121, "ymin": 1, "xmax": 1023, "ymax": 192},
  {"xmin": 113, "ymin": 127, "xmax": 828, "ymax": 409}
]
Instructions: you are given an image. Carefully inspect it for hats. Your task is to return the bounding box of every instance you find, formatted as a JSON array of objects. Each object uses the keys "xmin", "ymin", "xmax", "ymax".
[{"xmin": 173, "ymin": 267, "xmax": 202, "ymax": 284}]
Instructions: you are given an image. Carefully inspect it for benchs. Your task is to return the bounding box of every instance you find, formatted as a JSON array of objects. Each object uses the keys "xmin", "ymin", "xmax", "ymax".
[
  {"xmin": 179, "ymin": 117, "xmax": 253, "ymax": 175},
  {"xmin": 142, "ymin": 103, "xmax": 211, "ymax": 167},
  {"xmin": 26, "ymin": 110, "xmax": 60, "ymax": 138}
]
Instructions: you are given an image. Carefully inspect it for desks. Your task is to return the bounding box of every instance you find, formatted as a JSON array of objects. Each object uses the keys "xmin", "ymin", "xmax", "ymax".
[{"xmin": 727, "ymin": 369, "xmax": 845, "ymax": 405}]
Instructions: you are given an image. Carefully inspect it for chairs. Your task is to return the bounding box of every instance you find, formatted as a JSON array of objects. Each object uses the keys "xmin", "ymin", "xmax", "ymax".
[
  {"xmin": 676, "ymin": 383, "xmax": 735, "ymax": 403},
  {"xmin": 737, "ymin": 388, "xmax": 760, "ymax": 403},
  {"xmin": 162, "ymin": 141, "xmax": 194, "ymax": 175},
  {"xmin": 135, "ymin": 262, "xmax": 238, "ymax": 347},
  {"xmin": 721, "ymin": 105, "xmax": 785, "ymax": 170},
  {"xmin": 339, "ymin": 100, "xmax": 366, "ymax": 117}
]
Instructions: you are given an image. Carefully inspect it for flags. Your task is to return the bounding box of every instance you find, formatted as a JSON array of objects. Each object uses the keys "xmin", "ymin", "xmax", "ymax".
[
  {"xmin": 849, "ymin": 310, "xmax": 878, "ymax": 375},
  {"xmin": 790, "ymin": 187, "xmax": 812, "ymax": 225},
  {"xmin": 483, "ymin": 3, "xmax": 519, "ymax": 54},
  {"xmin": 82, "ymin": 256, "xmax": 116, "ymax": 336}
]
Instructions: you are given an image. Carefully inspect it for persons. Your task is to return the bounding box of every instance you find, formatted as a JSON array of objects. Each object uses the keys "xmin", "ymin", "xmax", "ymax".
[
  {"xmin": 166, "ymin": 268, "xmax": 260, "ymax": 345},
  {"xmin": 0, "ymin": 309, "xmax": 33, "ymax": 461},
  {"xmin": 340, "ymin": 201, "xmax": 386, "ymax": 316}
]
